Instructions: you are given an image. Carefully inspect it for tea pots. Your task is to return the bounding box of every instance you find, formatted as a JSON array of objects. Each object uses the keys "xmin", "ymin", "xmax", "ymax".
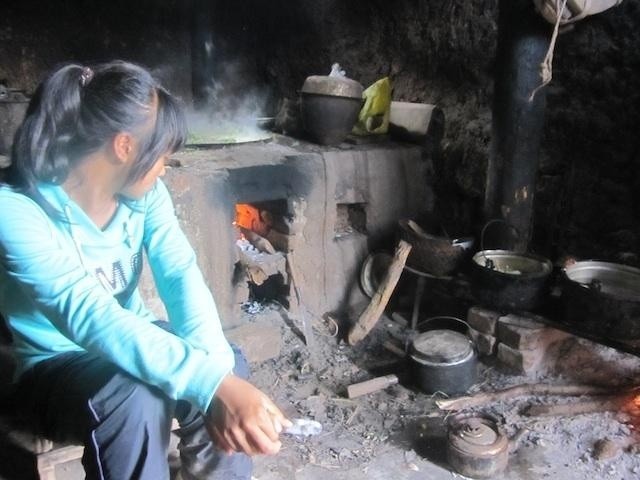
[
  {"xmin": 381, "ymin": 313, "xmax": 482, "ymax": 398},
  {"xmin": 443, "ymin": 409, "xmax": 531, "ymax": 480}
]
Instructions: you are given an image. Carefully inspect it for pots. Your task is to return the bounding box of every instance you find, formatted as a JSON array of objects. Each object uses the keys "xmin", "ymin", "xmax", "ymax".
[
  {"xmin": 394, "ymin": 212, "xmax": 476, "ymax": 276},
  {"xmin": 182, "ymin": 129, "xmax": 274, "ymax": 148},
  {"xmin": 558, "ymin": 255, "xmax": 640, "ymax": 340},
  {"xmin": 294, "ymin": 62, "xmax": 367, "ymax": 146}
]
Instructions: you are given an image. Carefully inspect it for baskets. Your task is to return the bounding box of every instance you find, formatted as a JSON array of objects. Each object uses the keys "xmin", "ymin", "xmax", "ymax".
[{"xmin": 396, "ymin": 200, "xmax": 475, "ymax": 277}]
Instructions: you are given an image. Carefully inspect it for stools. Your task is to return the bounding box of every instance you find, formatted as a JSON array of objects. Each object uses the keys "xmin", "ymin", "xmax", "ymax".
[{"xmin": 0, "ymin": 417, "xmax": 180, "ymax": 480}]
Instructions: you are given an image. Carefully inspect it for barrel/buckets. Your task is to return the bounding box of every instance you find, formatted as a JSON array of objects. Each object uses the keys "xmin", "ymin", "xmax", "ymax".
[{"xmin": 404, "ymin": 315, "xmax": 478, "ymax": 398}]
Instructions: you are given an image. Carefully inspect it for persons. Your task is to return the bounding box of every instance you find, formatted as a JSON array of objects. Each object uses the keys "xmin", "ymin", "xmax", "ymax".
[{"xmin": 0, "ymin": 59, "xmax": 292, "ymax": 480}]
